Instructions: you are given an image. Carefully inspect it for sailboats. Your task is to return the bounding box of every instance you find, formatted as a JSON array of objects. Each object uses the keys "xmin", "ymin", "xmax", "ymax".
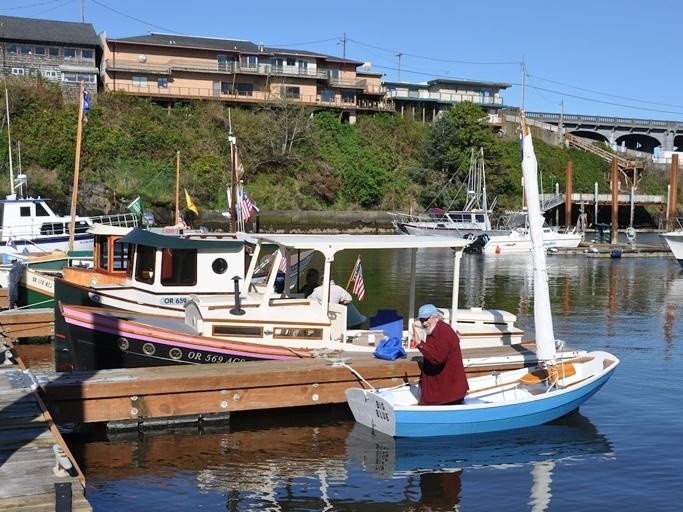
[
  {"xmin": 343, "ymin": 102, "xmax": 623, "ymax": 441},
  {"xmin": 347, "ymin": 408, "xmax": 618, "ymax": 512},
  {"xmin": 385, "ymin": 136, "xmax": 588, "ymax": 257}
]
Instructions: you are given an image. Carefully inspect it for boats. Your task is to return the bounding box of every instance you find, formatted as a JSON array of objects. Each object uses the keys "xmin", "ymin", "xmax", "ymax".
[
  {"xmin": 657, "ymin": 217, "xmax": 682, "ymax": 271},
  {"xmin": 52, "ymin": 230, "xmax": 525, "ymax": 372}
]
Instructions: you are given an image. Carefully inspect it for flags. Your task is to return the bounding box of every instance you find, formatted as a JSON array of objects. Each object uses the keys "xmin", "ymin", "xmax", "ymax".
[
  {"xmin": 184, "ymin": 189, "xmax": 199, "ymax": 216},
  {"xmin": 127, "ymin": 196, "xmax": 141, "ymax": 218},
  {"xmin": 83, "ymin": 91, "xmax": 91, "ymax": 125},
  {"xmin": 351, "ymin": 263, "xmax": 366, "ymax": 301},
  {"xmin": 227, "ymin": 147, "xmax": 253, "ymax": 222}
]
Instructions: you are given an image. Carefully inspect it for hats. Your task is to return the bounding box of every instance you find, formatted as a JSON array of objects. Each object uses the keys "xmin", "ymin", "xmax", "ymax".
[{"xmin": 416, "ymin": 302, "xmax": 440, "ymax": 321}]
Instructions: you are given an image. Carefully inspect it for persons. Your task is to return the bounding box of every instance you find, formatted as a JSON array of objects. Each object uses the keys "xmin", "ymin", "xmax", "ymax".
[
  {"xmin": 411, "ymin": 303, "xmax": 469, "ymax": 406},
  {"xmin": 306, "ymin": 275, "xmax": 353, "ymax": 306}
]
